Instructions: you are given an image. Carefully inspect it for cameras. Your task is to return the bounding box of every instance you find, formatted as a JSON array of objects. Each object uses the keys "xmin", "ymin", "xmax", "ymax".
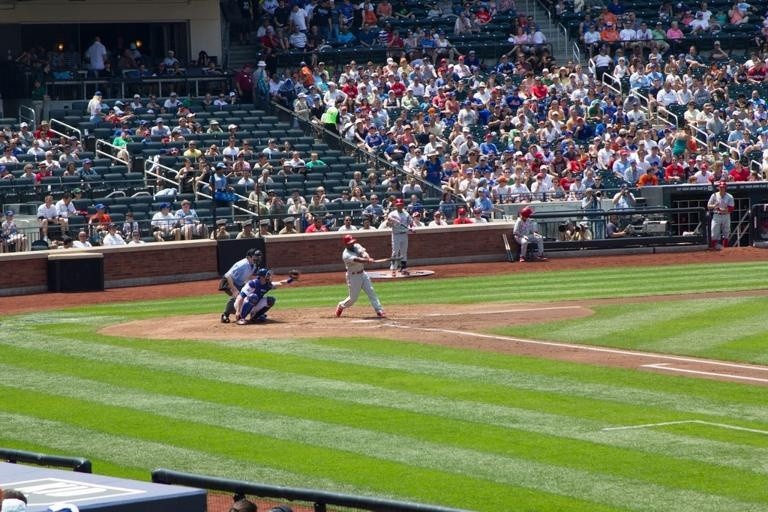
[
  {"xmin": 558, "ymin": 220, "xmax": 569, "ymax": 232},
  {"xmin": 575, "ymin": 223, "xmax": 588, "ymax": 232},
  {"xmin": 624, "ymin": 188, "xmax": 630, "ymax": 193},
  {"xmin": 591, "ymin": 191, "xmax": 602, "ymax": 198}
]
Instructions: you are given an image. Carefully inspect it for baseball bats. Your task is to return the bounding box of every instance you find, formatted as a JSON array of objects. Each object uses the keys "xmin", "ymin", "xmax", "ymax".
[
  {"xmin": 503, "ymin": 233, "xmax": 514, "ymax": 262},
  {"xmin": 374, "ymin": 257, "xmax": 404, "ymax": 263},
  {"xmin": 711, "ymin": 181, "xmax": 722, "ymax": 215},
  {"xmin": 388, "ymin": 218, "xmax": 416, "ymax": 233}
]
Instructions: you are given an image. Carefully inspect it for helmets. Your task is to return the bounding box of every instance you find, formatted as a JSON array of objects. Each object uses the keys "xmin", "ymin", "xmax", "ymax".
[{"xmin": 247, "ymin": 248, "xmax": 271, "ymax": 277}]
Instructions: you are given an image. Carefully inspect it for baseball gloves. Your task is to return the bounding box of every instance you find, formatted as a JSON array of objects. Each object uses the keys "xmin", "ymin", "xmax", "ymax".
[{"xmin": 289, "ymin": 270, "xmax": 300, "ymax": 280}]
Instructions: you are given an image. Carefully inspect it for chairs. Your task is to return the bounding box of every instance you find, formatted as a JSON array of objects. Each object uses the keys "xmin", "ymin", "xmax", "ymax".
[{"xmin": 0, "ymin": 2, "xmax": 768, "ymax": 264}]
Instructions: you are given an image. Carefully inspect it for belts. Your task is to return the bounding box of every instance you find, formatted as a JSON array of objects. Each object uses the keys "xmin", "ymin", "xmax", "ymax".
[{"xmin": 353, "ymin": 270, "xmax": 363, "ymax": 274}]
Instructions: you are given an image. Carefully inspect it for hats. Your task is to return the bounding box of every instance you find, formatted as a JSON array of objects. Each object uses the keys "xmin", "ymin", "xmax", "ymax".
[
  {"xmin": 156, "ymin": 117, "xmax": 163, "ymax": 124},
  {"xmin": 228, "ymin": 124, "xmax": 237, "ymax": 130},
  {"xmin": 344, "ymin": 235, "xmax": 356, "ymax": 244},
  {"xmin": 412, "ymin": 211, "xmax": 420, "ymax": 218},
  {"xmin": 0, "ymin": 0, "xmax": 768, "ymax": 228}
]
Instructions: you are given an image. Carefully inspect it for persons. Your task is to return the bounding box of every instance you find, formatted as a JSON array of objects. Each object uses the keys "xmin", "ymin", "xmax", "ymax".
[
  {"xmin": 217, "ymin": 249, "xmax": 273, "ymax": 323},
  {"xmin": 336, "ymin": 234, "xmax": 387, "ymax": 318},
  {"xmin": 234, "ymin": 269, "xmax": 299, "ymax": 325}
]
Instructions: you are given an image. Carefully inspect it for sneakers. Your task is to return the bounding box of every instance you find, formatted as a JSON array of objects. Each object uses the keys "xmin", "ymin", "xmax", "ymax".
[
  {"xmin": 221, "ymin": 315, "xmax": 247, "ymax": 325},
  {"xmin": 377, "ymin": 312, "xmax": 385, "ymax": 317},
  {"xmin": 335, "ymin": 306, "xmax": 343, "ymax": 317}
]
